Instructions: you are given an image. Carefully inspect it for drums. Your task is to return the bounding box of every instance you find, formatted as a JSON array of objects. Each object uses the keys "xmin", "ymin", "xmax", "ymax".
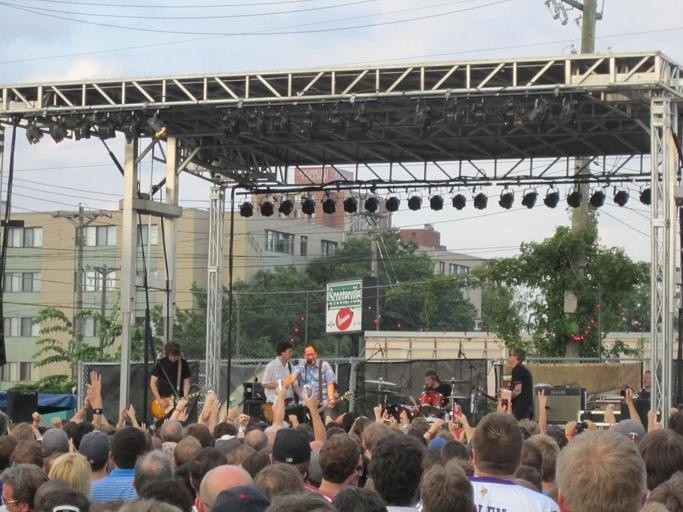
[
  {"xmin": 420, "ymin": 391, "xmax": 445, "ymax": 416},
  {"xmin": 387, "ymin": 403, "xmax": 412, "ymax": 419}
]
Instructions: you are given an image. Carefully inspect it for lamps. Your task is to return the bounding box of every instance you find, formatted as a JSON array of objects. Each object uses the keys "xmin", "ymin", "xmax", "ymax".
[
  {"xmin": 383, "ymin": 188, "xmax": 403, "ymax": 214},
  {"xmin": 276, "ymin": 191, "xmax": 294, "ymax": 218},
  {"xmin": 319, "ymin": 189, "xmax": 340, "ymax": 217},
  {"xmin": 612, "ymin": 183, "xmax": 632, "ymax": 208},
  {"xmin": 589, "ymin": 183, "xmax": 607, "ymax": 210},
  {"xmin": 470, "ymin": 185, "xmax": 490, "ymax": 211},
  {"xmin": 413, "ymin": 98, "xmax": 580, "ymax": 136},
  {"xmin": 519, "ymin": 184, "xmax": 539, "ymax": 210},
  {"xmin": 213, "ymin": 100, "xmax": 379, "ymax": 141},
  {"xmin": 340, "ymin": 188, "xmax": 360, "ymax": 215},
  {"xmin": 406, "ymin": 185, "xmax": 425, "ymax": 213},
  {"xmin": 565, "ymin": 184, "xmax": 585, "ymax": 210},
  {"xmin": 426, "ymin": 186, "xmax": 446, "ymax": 212},
  {"xmin": 497, "ymin": 183, "xmax": 517, "ymax": 212},
  {"xmin": 543, "ymin": 184, "xmax": 562, "ymax": 211},
  {"xmin": 362, "ymin": 186, "xmax": 382, "ymax": 214},
  {"xmin": 298, "ymin": 191, "xmax": 318, "ymax": 217},
  {"xmin": 638, "ymin": 181, "xmax": 654, "ymax": 207},
  {"xmin": 238, "ymin": 193, "xmax": 255, "ymax": 218},
  {"xmin": 26, "ymin": 105, "xmax": 173, "ymax": 145},
  {"xmin": 446, "ymin": 184, "xmax": 468, "ymax": 212},
  {"xmin": 258, "ymin": 192, "xmax": 276, "ymax": 219}
]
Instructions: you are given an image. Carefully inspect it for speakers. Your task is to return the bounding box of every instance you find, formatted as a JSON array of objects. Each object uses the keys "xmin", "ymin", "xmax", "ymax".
[
  {"xmin": 9, "ymin": 391, "xmax": 37, "ymax": 425},
  {"xmin": 621, "ymin": 398, "xmax": 651, "ymax": 428}
]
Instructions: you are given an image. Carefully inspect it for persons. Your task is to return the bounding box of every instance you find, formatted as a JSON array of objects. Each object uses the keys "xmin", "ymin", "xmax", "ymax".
[{"xmin": 0, "ymin": 340, "xmax": 683, "ymax": 512}]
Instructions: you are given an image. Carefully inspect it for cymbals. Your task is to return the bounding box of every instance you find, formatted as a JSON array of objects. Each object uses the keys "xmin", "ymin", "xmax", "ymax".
[
  {"xmin": 365, "ymin": 379, "xmax": 396, "ymax": 385},
  {"xmin": 447, "ymin": 395, "xmax": 469, "ymax": 398},
  {"xmin": 369, "ymin": 389, "xmax": 399, "ymax": 396},
  {"xmin": 443, "ymin": 379, "xmax": 468, "ymax": 383}
]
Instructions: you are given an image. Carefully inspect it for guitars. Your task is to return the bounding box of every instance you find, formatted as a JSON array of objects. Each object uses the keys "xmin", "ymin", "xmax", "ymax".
[
  {"xmin": 262, "ymin": 396, "xmax": 303, "ymax": 423},
  {"xmin": 303, "ymin": 389, "xmax": 354, "ymax": 428},
  {"xmin": 150, "ymin": 391, "xmax": 201, "ymax": 420}
]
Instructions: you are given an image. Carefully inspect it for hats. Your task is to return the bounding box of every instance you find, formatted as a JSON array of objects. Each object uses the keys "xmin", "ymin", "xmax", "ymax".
[
  {"xmin": 614, "ymin": 419, "xmax": 646, "ymax": 443},
  {"xmin": 79, "ymin": 432, "xmax": 109, "ymax": 464},
  {"xmin": 273, "ymin": 429, "xmax": 310, "ymax": 464},
  {"xmin": 213, "ymin": 486, "xmax": 270, "ymax": 512}
]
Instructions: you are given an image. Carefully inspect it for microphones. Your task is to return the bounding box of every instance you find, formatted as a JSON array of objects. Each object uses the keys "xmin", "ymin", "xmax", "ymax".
[
  {"xmin": 457, "ymin": 342, "xmax": 463, "ymax": 358},
  {"xmin": 378, "ymin": 345, "xmax": 385, "ymax": 356},
  {"xmin": 303, "ymin": 359, "xmax": 314, "ymax": 367}
]
